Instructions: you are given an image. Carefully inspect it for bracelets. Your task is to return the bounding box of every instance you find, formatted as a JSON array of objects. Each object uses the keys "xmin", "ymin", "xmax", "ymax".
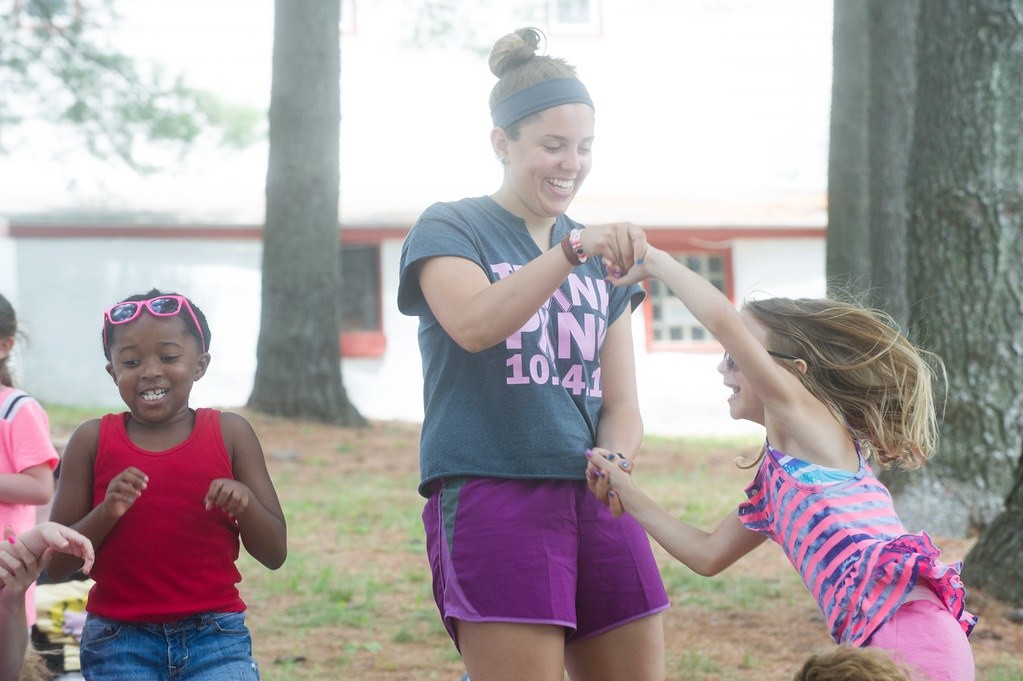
[
  {"xmin": 572, "ymin": 229, "xmax": 591, "ymax": 263},
  {"xmin": 561, "ymin": 234, "xmax": 582, "ymax": 266},
  {"xmin": 618, "ymin": 453, "xmax": 625, "ymax": 459}
]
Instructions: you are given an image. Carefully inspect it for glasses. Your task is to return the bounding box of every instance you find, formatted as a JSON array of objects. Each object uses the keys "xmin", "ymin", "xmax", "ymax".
[
  {"xmin": 724, "ymin": 350, "xmax": 811, "ymax": 375},
  {"xmin": 104, "ymin": 295, "xmax": 205, "ymax": 363}
]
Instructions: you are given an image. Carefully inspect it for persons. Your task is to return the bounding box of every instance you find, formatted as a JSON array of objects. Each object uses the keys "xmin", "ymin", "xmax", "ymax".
[
  {"xmin": 585, "ymin": 242, "xmax": 977, "ymax": 681},
  {"xmin": 45, "ymin": 288, "xmax": 288, "ymax": 680},
  {"xmin": 795, "ymin": 644, "xmax": 912, "ymax": 681},
  {"xmin": 0, "ymin": 521, "xmax": 95, "ymax": 681},
  {"xmin": 399, "ymin": 33, "xmax": 670, "ymax": 681},
  {"xmin": 0, "ymin": 296, "xmax": 61, "ymax": 629}
]
